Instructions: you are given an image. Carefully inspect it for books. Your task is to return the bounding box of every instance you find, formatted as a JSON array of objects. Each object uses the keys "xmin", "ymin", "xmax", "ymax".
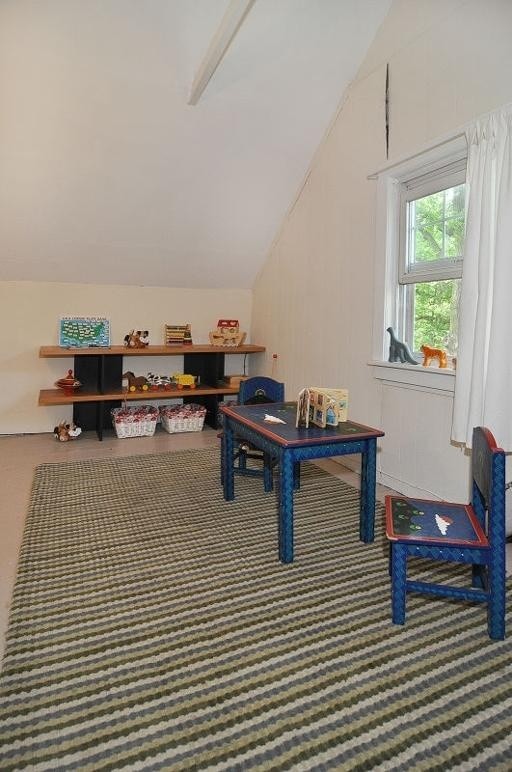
[{"xmin": 293, "ymin": 385, "xmax": 350, "ymax": 429}]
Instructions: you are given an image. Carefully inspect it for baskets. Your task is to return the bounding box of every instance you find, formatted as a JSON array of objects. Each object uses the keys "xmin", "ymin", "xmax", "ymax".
[
  {"xmin": 110, "ymin": 405, "xmax": 159, "ymax": 438},
  {"xmin": 159, "ymin": 403, "xmax": 207, "ymax": 434}
]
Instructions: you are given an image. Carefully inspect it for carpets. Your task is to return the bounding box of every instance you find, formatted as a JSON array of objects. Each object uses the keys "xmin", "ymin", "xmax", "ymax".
[{"xmin": 0, "ymin": 437, "xmax": 512, "ymax": 772}]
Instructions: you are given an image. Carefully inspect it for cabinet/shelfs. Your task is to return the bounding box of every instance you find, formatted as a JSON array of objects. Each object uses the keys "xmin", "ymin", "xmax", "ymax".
[{"xmin": 37, "ymin": 339, "xmax": 266, "ymax": 443}]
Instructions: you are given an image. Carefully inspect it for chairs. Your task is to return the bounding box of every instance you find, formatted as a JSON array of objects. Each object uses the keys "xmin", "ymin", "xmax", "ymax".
[
  {"xmin": 215, "ymin": 374, "xmax": 286, "ymax": 495},
  {"xmin": 383, "ymin": 421, "xmax": 510, "ymax": 642}
]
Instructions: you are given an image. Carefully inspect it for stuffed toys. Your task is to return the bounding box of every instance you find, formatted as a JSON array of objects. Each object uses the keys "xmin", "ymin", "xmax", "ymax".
[
  {"xmin": 53, "ymin": 419, "xmax": 83, "ymax": 443},
  {"xmin": 124, "ymin": 329, "xmax": 152, "ymax": 350}
]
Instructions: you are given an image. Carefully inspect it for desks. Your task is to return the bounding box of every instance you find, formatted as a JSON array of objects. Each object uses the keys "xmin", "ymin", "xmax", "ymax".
[{"xmin": 215, "ymin": 400, "xmax": 388, "ymax": 565}]
